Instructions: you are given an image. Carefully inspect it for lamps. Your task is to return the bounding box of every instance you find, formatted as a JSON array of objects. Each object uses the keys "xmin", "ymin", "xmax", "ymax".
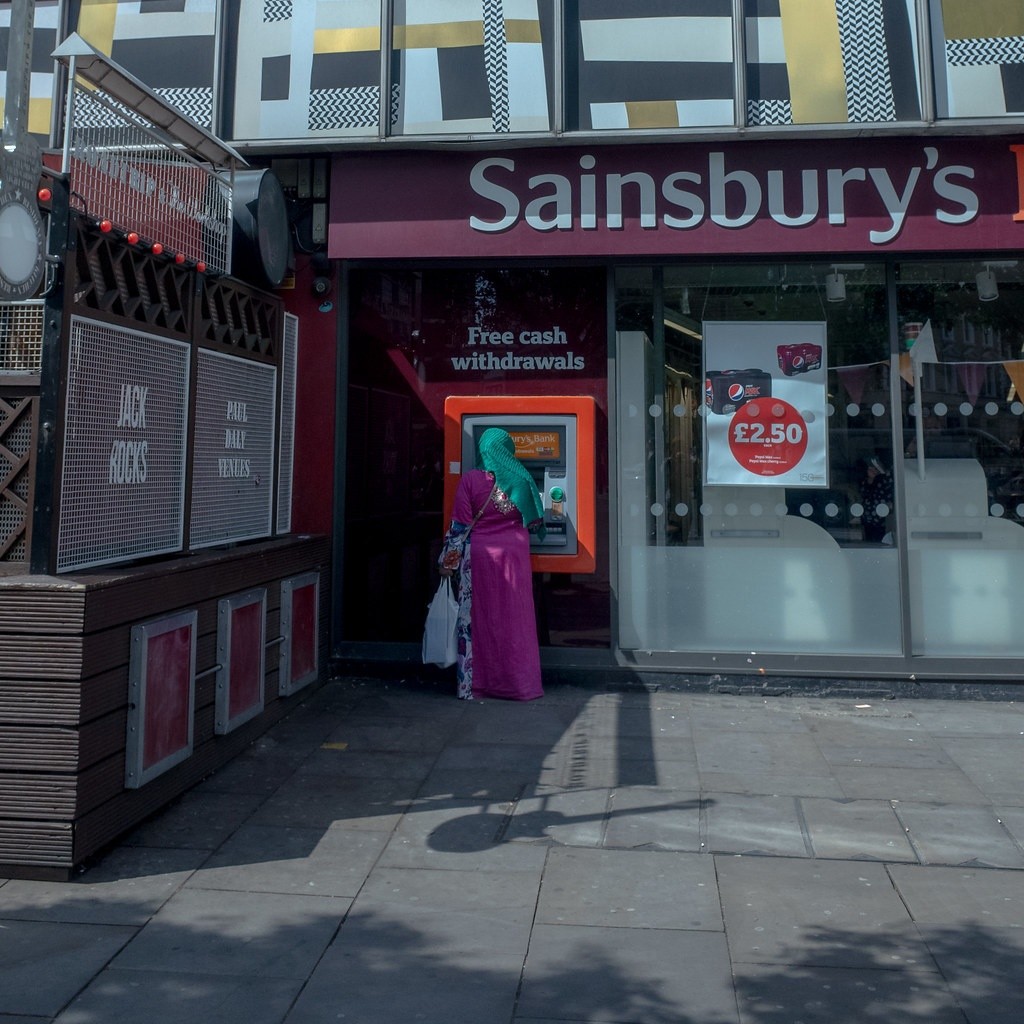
[
  {"xmin": 976, "ymin": 261, "xmax": 1018, "ymax": 302},
  {"xmin": 826, "ymin": 264, "xmax": 865, "ymax": 302},
  {"xmin": 652, "ymin": 306, "xmax": 703, "ymax": 341}
]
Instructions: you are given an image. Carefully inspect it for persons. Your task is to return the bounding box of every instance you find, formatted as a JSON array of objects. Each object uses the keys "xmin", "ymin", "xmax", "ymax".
[
  {"xmin": 845, "ymin": 414, "xmax": 973, "ymax": 544},
  {"xmin": 437, "ymin": 424, "xmax": 545, "ymax": 701}
]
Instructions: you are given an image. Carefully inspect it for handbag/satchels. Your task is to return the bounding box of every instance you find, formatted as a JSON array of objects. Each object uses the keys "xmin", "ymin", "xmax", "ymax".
[
  {"xmin": 437, "ymin": 535, "xmax": 464, "ymax": 570},
  {"xmin": 421, "ymin": 576, "xmax": 459, "ymax": 669}
]
